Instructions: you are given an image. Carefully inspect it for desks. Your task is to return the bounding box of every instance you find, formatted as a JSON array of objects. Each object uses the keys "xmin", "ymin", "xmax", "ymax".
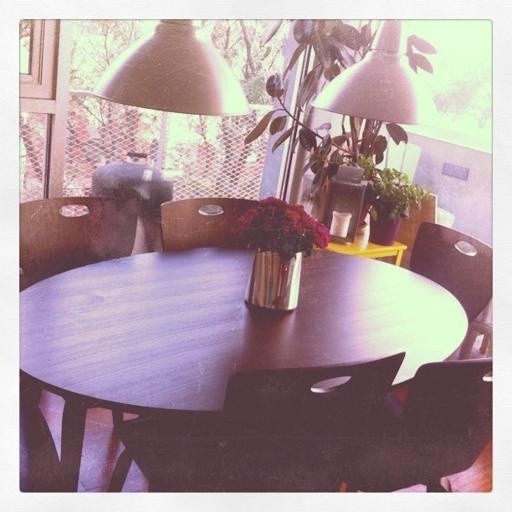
[{"xmin": 327, "ymin": 238, "xmax": 407, "ymax": 266}]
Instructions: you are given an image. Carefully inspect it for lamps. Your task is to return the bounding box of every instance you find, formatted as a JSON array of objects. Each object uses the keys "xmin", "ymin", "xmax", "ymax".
[{"xmin": 92, "ymin": 19, "xmax": 251, "ymax": 120}]
